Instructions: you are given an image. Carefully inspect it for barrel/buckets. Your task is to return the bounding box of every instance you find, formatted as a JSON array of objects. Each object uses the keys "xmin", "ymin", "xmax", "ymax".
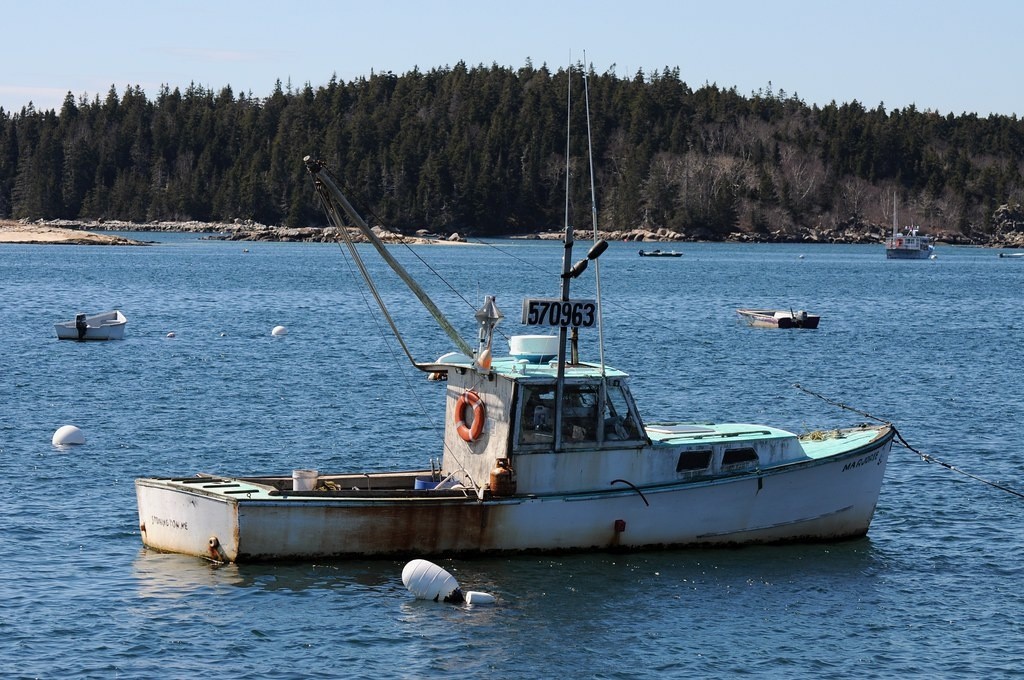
[
  {"xmin": 292, "ymin": 468, "xmax": 319, "ymax": 489},
  {"xmin": 414, "ymin": 477, "xmax": 461, "ymax": 488}
]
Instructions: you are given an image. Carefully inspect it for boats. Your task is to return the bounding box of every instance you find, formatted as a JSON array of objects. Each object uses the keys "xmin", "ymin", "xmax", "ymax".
[
  {"xmin": 735, "ymin": 307, "xmax": 821, "ymax": 330},
  {"xmin": 885, "ymin": 220, "xmax": 935, "ymax": 259},
  {"xmin": 54, "ymin": 309, "xmax": 127, "ymax": 340},
  {"xmin": 132, "ymin": 51, "xmax": 898, "ymax": 571},
  {"xmin": 638, "ymin": 249, "xmax": 684, "ymax": 258},
  {"xmin": 997, "ymin": 253, "xmax": 1024, "ymax": 258}
]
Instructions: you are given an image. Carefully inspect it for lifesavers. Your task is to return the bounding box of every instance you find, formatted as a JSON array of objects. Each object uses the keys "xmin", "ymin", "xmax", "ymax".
[
  {"xmin": 454, "ymin": 390, "xmax": 486, "ymax": 443},
  {"xmin": 896, "ymin": 238, "xmax": 902, "ymax": 247}
]
